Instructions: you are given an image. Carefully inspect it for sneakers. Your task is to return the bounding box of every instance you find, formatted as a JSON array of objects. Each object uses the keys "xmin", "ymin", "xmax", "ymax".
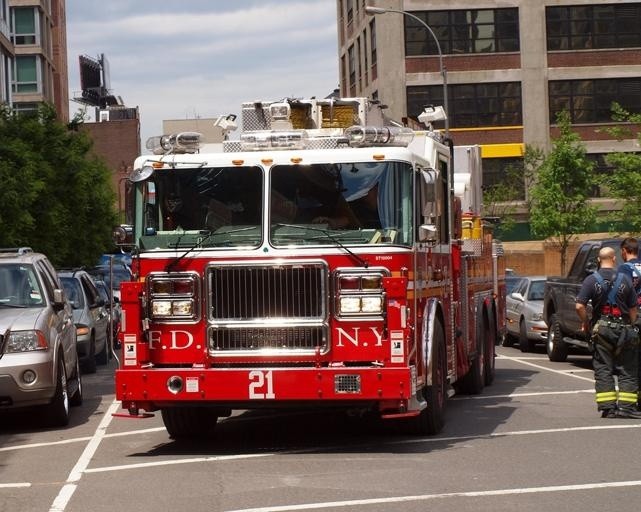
[
  {"xmin": 617, "ymin": 408, "xmax": 641, "ymax": 420},
  {"xmin": 602, "ymin": 408, "xmax": 616, "ymax": 419}
]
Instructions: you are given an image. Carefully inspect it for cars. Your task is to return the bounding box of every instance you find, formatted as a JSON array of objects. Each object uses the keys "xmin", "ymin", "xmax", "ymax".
[
  {"xmin": 0, "ymin": 244, "xmax": 131, "ymax": 430},
  {"xmin": 499, "ymin": 238, "xmax": 553, "ymax": 363}
]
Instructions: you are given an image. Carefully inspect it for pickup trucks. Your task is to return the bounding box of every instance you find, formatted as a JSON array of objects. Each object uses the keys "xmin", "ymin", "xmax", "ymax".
[{"xmin": 543, "ymin": 238, "xmax": 641, "ymax": 363}]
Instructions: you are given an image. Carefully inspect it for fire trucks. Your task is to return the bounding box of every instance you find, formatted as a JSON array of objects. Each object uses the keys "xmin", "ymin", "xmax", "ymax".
[{"xmin": 108, "ymin": 96, "xmax": 508, "ymax": 440}]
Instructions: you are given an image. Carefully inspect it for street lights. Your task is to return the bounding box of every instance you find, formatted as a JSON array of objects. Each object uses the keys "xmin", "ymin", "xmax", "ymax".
[{"xmin": 363, "ymin": 6, "xmax": 452, "ymax": 139}]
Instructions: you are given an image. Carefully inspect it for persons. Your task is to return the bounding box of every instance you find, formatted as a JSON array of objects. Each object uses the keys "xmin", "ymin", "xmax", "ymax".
[
  {"xmin": 577, "ymin": 245, "xmax": 640, "ymax": 418},
  {"xmin": 310, "ymin": 181, "xmax": 385, "ymax": 230},
  {"xmin": 616, "ymin": 238, "xmax": 641, "ymax": 414}
]
